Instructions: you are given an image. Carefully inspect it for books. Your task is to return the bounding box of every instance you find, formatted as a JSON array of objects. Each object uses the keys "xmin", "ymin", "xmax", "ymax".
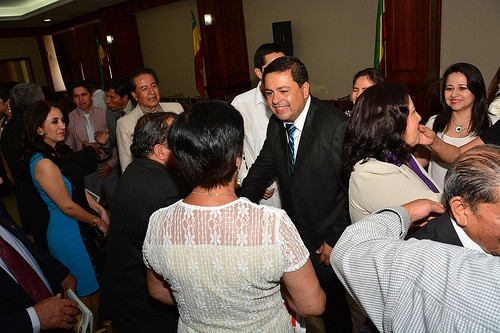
[{"xmin": 64, "ymin": 288, "xmax": 94, "ymax": 333}]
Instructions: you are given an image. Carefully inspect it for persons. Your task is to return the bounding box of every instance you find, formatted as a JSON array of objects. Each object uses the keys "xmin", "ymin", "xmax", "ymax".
[
  {"xmin": 67, "ymin": 81, "xmax": 120, "ymax": 203},
  {"xmin": 230, "ymin": 43, "xmax": 282, "ymax": 211},
  {"xmin": 404, "ymin": 144, "xmax": 500, "ymax": 256},
  {"xmin": 418, "ymin": 67, "xmax": 500, "ymax": 165},
  {"xmin": 340, "ymin": 82, "xmax": 442, "ymax": 224},
  {"xmin": 88, "ymin": 79, "xmax": 108, "ymax": 109},
  {"xmin": 116, "ymin": 68, "xmax": 184, "ymax": 175},
  {"xmin": 237, "ymin": 56, "xmax": 353, "ymax": 333},
  {"xmin": 413, "ymin": 63, "xmax": 486, "ymax": 190},
  {"xmin": 0, "ymin": 177, "xmax": 79, "ymax": 333},
  {"xmin": 93, "ymin": 77, "xmax": 137, "ymax": 150},
  {"xmin": 344, "ymin": 68, "xmax": 381, "ymax": 118},
  {"xmin": 331, "ymin": 199, "xmax": 500, "ymax": 333},
  {"xmin": 21, "ymin": 100, "xmax": 110, "ymax": 333},
  {"xmin": 97, "ymin": 112, "xmax": 193, "ymax": 333},
  {"xmin": 142, "ymin": 101, "xmax": 326, "ymax": 333},
  {"xmin": 0, "ymin": 82, "xmax": 102, "ymax": 252}
]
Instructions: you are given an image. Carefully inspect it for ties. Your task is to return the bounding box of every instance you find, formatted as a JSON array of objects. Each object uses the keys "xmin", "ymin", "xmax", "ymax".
[
  {"xmin": 285, "ymin": 124, "xmax": 297, "ymax": 177},
  {"xmin": 0, "ymin": 235, "xmax": 54, "ymax": 304},
  {"xmin": 83, "ymin": 114, "xmax": 96, "ymax": 143},
  {"xmin": 120, "ymin": 110, "xmax": 125, "ymax": 117}
]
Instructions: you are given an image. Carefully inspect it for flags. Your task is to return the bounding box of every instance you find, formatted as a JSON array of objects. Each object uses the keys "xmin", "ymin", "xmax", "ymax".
[
  {"xmin": 374, "ymin": 0, "xmax": 387, "ymax": 72},
  {"xmin": 191, "ymin": 14, "xmax": 206, "ymax": 98},
  {"xmin": 95, "ymin": 34, "xmax": 109, "ymax": 79}
]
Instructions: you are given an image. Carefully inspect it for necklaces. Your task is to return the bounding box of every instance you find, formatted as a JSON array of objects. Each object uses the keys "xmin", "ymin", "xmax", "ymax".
[
  {"xmin": 452, "ymin": 120, "xmax": 461, "ymax": 132},
  {"xmin": 192, "ymin": 191, "xmax": 236, "ymax": 197}
]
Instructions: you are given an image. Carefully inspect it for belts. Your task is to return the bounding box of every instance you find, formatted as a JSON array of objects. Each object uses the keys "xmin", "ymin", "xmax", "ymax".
[{"xmin": 97, "ymin": 153, "xmax": 112, "ymax": 164}]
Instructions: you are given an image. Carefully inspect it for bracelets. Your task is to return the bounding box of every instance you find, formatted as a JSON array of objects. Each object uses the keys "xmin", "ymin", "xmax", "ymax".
[{"xmin": 91, "ymin": 218, "xmax": 97, "ymax": 226}]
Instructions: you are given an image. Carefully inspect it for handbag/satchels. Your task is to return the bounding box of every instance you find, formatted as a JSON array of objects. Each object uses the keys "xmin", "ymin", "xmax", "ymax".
[{"xmin": 72, "ymin": 184, "xmax": 104, "ymax": 242}]
